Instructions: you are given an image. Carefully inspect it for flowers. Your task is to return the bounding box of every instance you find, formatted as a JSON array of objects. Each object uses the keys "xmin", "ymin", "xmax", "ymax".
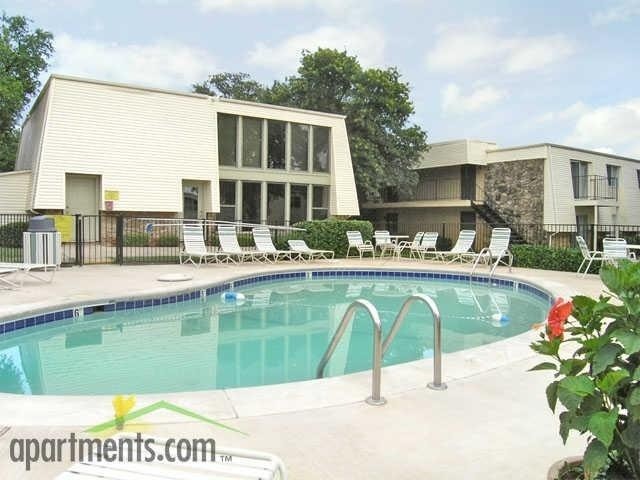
[{"xmin": 527, "ymin": 255, "xmax": 640, "ymax": 480}]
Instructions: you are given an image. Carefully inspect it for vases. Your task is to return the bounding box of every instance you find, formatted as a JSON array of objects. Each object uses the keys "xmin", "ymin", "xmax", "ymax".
[{"xmin": 546, "ymin": 455, "xmax": 609, "ymax": 480}]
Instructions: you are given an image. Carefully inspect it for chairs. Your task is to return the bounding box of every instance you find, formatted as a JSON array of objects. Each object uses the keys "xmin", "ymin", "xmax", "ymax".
[
  {"xmin": 178, "ymin": 224, "xmax": 335, "ymax": 272},
  {"xmin": 0, "ymin": 262, "xmax": 57, "ymax": 292},
  {"xmin": 346, "ymin": 227, "xmax": 512, "ymax": 275},
  {"xmin": 575, "ymin": 235, "xmax": 637, "ymax": 278}
]
